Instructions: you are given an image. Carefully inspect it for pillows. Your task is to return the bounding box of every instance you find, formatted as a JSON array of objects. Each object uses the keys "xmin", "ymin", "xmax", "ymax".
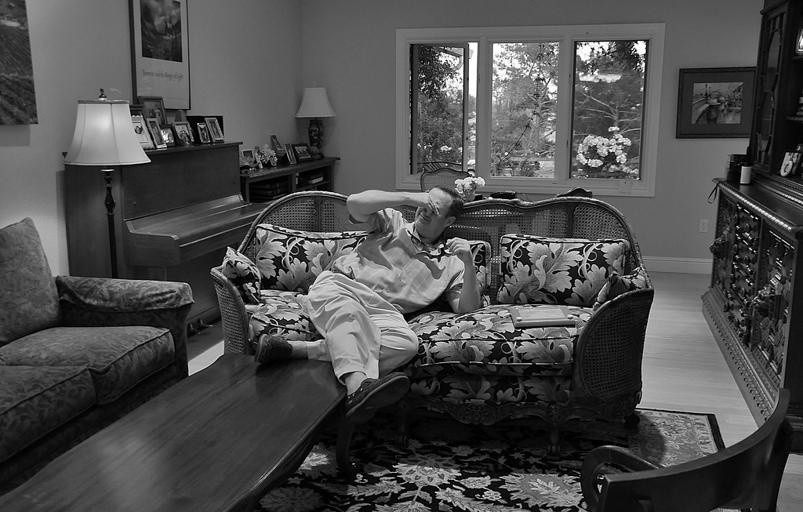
[
  {"xmin": 444, "ymin": 240, "xmax": 491, "ymax": 308},
  {"xmin": 496, "ymin": 233, "xmax": 630, "ymax": 307},
  {"xmin": 221, "ymin": 246, "xmax": 261, "ymax": 305},
  {"xmin": 591, "ymin": 267, "xmax": 647, "ymax": 315},
  {"xmin": 254, "ymin": 224, "xmax": 369, "ymax": 292}
]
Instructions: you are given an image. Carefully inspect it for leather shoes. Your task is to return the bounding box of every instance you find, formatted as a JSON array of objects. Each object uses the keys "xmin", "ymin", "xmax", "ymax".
[
  {"xmin": 254, "ymin": 333, "xmax": 294, "ymax": 364},
  {"xmin": 343, "ymin": 371, "xmax": 411, "ymax": 425}
]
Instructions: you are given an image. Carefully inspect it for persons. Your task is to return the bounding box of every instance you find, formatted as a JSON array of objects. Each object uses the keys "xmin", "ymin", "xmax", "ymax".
[
  {"xmin": 255, "ymin": 184, "xmax": 481, "ymax": 426},
  {"xmin": 154, "ymin": 104, "xmax": 164, "ymax": 126},
  {"xmin": 135, "ymin": 125, "xmax": 141, "ymax": 134}
]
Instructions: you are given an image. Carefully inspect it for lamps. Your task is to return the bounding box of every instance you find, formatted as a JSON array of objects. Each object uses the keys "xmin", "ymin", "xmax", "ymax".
[
  {"xmin": 63, "ymin": 88, "xmax": 151, "ymax": 278},
  {"xmin": 295, "ymin": 87, "xmax": 336, "ymax": 160}
]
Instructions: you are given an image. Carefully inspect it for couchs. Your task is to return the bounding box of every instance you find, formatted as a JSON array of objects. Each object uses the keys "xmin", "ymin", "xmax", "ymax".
[
  {"xmin": 209, "ymin": 191, "xmax": 654, "ymax": 456},
  {"xmin": 0, "ymin": 216, "xmax": 195, "ymax": 498}
]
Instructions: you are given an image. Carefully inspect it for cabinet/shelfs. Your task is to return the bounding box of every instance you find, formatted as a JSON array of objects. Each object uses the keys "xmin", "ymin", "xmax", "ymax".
[{"xmin": 701, "ymin": 0, "xmax": 803, "ymax": 455}]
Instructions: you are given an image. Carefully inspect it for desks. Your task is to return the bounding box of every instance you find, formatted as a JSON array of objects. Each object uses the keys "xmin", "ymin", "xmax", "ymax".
[
  {"xmin": 241, "ymin": 157, "xmax": 341, "ymax": 205},
  {"xmin": 404, "ymin": 209, "xmax": 523, "ymax": 255},
  {"xmin": 0, "ymin": 352, "xmax": 359, "ymax": 512}
]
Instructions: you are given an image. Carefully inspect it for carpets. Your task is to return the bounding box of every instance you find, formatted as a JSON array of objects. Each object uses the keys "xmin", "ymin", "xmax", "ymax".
[{"xmin": 250, "ymin": 408, "xmax": 750, "ymax": 512}]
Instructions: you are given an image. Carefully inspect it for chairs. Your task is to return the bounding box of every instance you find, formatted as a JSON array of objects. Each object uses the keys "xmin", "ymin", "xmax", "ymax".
[
  {"xmin": 528, "ymin": 187, "xmax": 592, "ymax": 238},
  {"xmin": 579, "ymin": 389, "xmax": 791, "ymax": 512},
  {"xmin": 420, "ymin": 168, "xmax": 474, "ymax": 193}
]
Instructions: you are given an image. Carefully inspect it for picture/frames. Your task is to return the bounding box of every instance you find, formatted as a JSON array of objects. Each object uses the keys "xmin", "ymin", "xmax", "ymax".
[
  {"xmin": 284, "ymin": 143, "xmax": 297, "ymax": 165},
  {"xmin": 509, "ymin": 306, "xmax": 575, "ymax": 328},
  {"xmin": 292, "ymin": 144, "xmax": 313, "ymax": 163},
  {"xmin": 129, "ymin": 96, "xmax": 225, "ymax": 149},
  {"xmin": 128, "ymin": 0, "xmax": 191, "ymax": 110},
  {"xmin": 676, "ymin": 67, "xmax": 757, "ymax": 138}
]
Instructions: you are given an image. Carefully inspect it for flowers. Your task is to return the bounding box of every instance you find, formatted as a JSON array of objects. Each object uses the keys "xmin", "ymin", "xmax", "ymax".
[{"xmin": 453, "ymin": 177, "xmax": 486, "ymax": 201}]
